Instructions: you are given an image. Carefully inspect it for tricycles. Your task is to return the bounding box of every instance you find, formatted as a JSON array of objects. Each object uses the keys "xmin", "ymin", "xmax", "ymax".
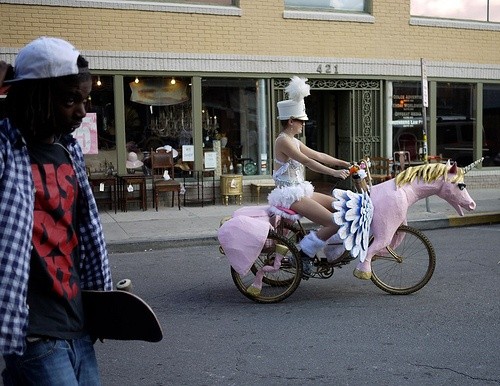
[{"xmin": 219, "ymin": 155, "xmax": 436, "ymax": 304}]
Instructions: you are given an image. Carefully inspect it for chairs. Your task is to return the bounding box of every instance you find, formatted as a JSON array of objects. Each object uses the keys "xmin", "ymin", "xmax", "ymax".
[
  {"xmin": 365, "ymin": 156, "xmax": 392, "ymax": 184},
  {"xmin": 394, "ymin": 151, "xmax": 410, "ymax": 178},
  {"xmin": 151, "ymin": 151, "xmax": 181, "ymax": 212}
]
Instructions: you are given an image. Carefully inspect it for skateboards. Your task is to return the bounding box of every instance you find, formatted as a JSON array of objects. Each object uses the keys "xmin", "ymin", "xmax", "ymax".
[{"xmin": 81, "ymin": 278, "xmax": 164, "ymax": 343}]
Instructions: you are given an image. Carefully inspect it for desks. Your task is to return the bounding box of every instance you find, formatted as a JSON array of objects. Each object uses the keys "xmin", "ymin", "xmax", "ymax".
[
  {"xmin": 184, "ymin": 168, "xmax": 216, "ymax": 208},
  {"xmin": 88, "ymin": 174, "xmax": 117, "ymax": 214},
  {"xmin": 251, "ymin": 183, "xmax": 276, "ymax": 205},
  {"xmin": 119, "ymin": 174, "xmax": 147, "ymax": 213}
]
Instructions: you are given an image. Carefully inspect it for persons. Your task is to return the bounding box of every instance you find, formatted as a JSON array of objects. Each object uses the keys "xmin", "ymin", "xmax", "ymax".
[
  {"xmin": 0, "ymin": 36, "xmax": 113, "ymax": 386},
  {"xmin": 268, "ymin": 72, "xmax": 371, "ymax": 277}
]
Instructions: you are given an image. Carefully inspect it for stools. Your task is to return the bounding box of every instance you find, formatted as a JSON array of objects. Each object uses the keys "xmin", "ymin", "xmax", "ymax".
[{"xmin": 221, "ymin": 174, "xmax": 242, "ymax": 206}]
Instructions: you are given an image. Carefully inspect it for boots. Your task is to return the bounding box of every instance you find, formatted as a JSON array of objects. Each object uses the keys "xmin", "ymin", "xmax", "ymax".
[
  {"xmin": 290, "ymin": 230, "xmax": 328, "ymax": 279},
  {"xmin": 323, "ymin": 233, "xmax": 359, "ymax": 264}
]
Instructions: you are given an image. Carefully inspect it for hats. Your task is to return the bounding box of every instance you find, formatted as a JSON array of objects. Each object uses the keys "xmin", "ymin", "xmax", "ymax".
[
  {"xmin": 155, "ymin": 145, "xmax": 179, "ymax": 158},
  {"xmin": 125, "ymin": 152, "xmax": 144, "ymax": 168},
  {"xmin": 2, "ymin": 37, "xmax": 90, "ymax": 83},
  {"xmin": 277, "ymin": 76, "xmax": 311, "ymax": 120}
]
